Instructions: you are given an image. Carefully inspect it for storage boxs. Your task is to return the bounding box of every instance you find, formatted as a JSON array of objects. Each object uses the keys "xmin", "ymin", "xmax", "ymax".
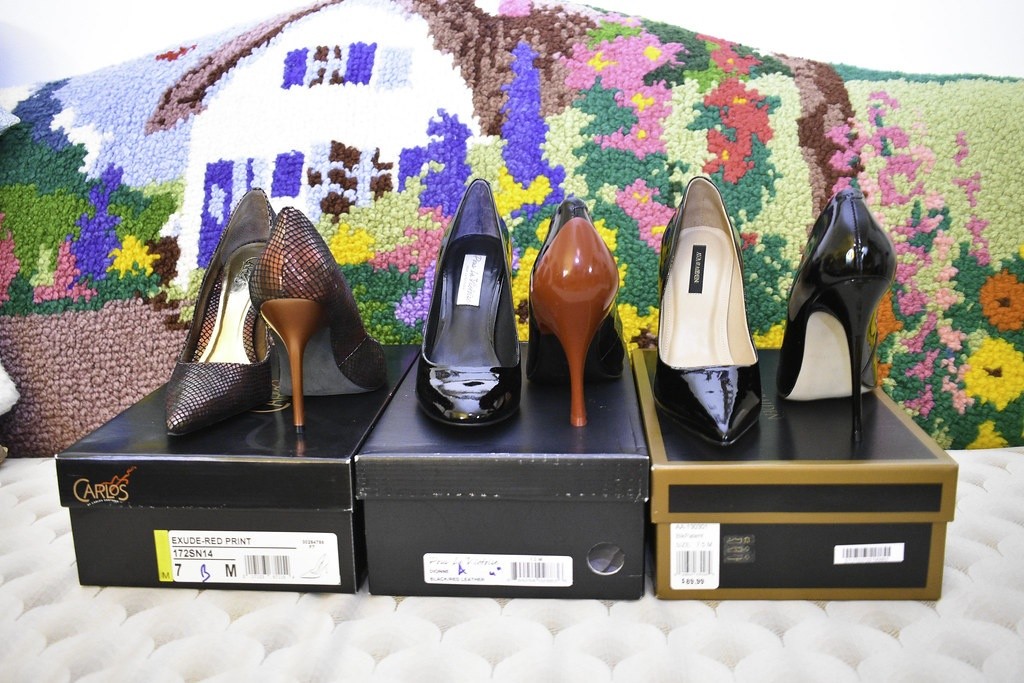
[
  {"xmin": 633, "ymin": 343, "xmax": 962, "ymax": 602},
  {"xmin": 353, "ymin": 336, "xmax": 650, "ymax": 602},
  {"xmin": 52, "ymin": 346, "xmax": 421, "ymax": 592}
]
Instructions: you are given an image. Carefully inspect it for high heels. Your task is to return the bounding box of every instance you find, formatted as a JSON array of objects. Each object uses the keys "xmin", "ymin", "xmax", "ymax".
[
  {"xmin": 163, "ymin": 189, "xmax": 277, "ymax": 436},
  {"xmin": 527, "ymin": 196, "xmax": 623, "ymax": 429},
  {"xmin": 416, "ymin": 180, "xmax": 520, "ymax": 432},
  {"xmin": 779, "ymin": 191, "xmax": 896, "ymax": 446},
  {"xmin": 653, "ymin": 177, "xmax": 763, "ymax": 448},
  {"xmin": 250, "ymin": 207, "xmax": 387, "ymax": 435}
]
